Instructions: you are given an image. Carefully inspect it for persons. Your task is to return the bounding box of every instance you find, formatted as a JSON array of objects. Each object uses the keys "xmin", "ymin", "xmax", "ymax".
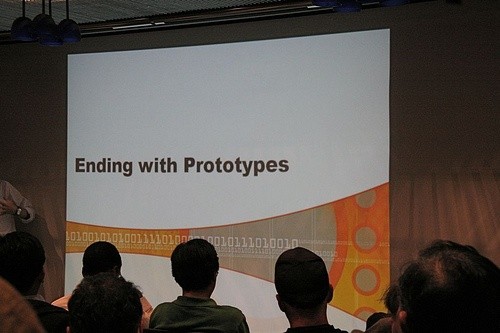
[
  {"xmin": 0, "ymin": 179, "xmax": 35, "ymax": 240},
  {"xmin": 0, "ymin": 231, "xmax": 500, "ymax": 333}
]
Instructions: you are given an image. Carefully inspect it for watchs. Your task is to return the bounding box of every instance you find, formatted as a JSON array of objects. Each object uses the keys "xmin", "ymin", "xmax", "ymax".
[{"xmin": 16, "ymin": 206, "xmax": 22, "ymax": 216}]
